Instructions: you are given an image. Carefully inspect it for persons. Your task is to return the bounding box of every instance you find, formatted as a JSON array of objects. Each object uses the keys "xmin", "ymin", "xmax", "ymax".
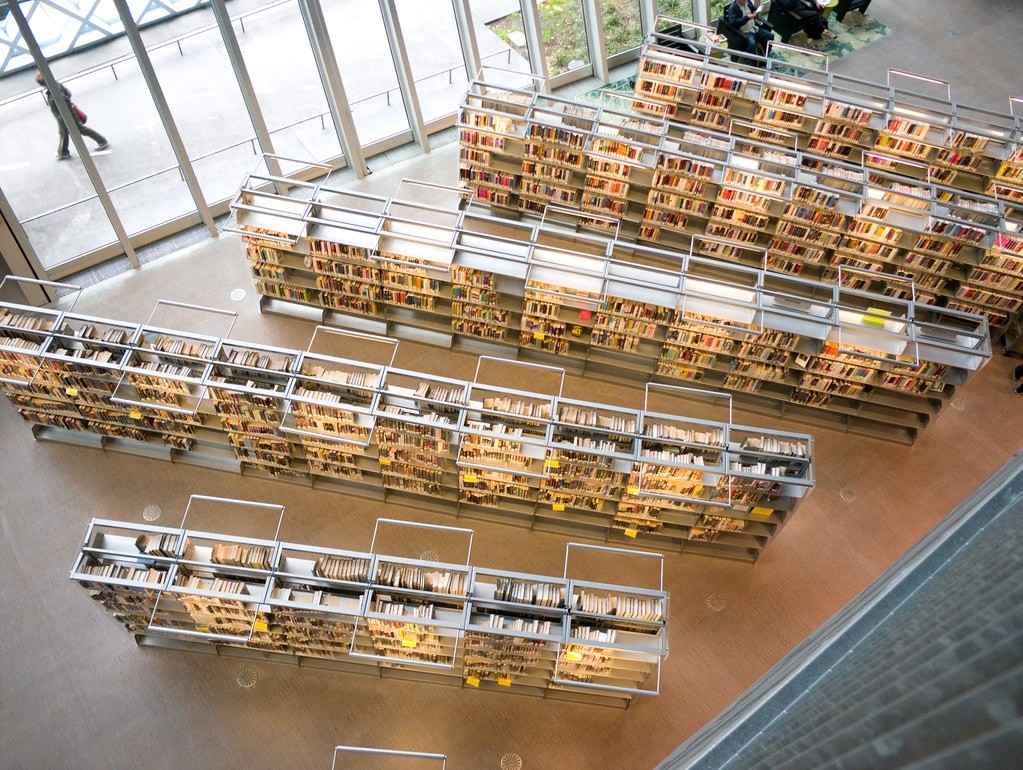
[
  {"xmin": 1013, "ymin": 363, "xmax": 1023, "ymax": 394},
  {"xmin": 729, "ymin": 0, "xmax": 775, "ymax": 72},
  {"xmin": 36, "ymin": 73, "xmax": 108, "ymax": 161},
  {"xmin": 788, "ymin": 0, "xmax": 837, "ymax": 51}
]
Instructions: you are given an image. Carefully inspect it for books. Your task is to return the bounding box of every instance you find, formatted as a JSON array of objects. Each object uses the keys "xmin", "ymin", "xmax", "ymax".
[
  {"xmin": 459, "ymin": 89, "xmax": 1023, "ymax": 327},
  {"xmin": 0, "ymin": 308, "xmax": 808, "ymax": 544},
  {"xmin": 754, "ymin": 4, "xmax": 764, "ymax": 15},
  {"xmin": 369, "ymin": 601, "xmax": 448, "ymax": 668},
  {"xmin": 463, "ymin": 614, "xmax": 550, "ymax": 680},
  {"xmin": 495, "ymin": 578, "xmax": 566, "ymax": 609},
  {"xmin": 240, "ymin": 222, "xmax": 950, "ymax": 408},
  {"xmin": 549, "ymin": 590, "xmax": 661, "ymax": 688},
  {"xmin": 209, "ymin": 544, "xmax": 281, "ymax": 571},
  {"xmin": 138, "ymin": 534, "xmax": 189, "ymax": 559},
  {"xmin": 77, "ymin": 565, "xmax": 365, "ymax": 658},
  {"xmin": 704, "ymin": 31, "xmax": 728, "ymax": 46},
  {"xmin": 311, "ymin": 555, "xmax": 467, "ymax": 608},
  {"xmin": 633, "ymin": 55, "xmax": 807, "ymax": 128}
]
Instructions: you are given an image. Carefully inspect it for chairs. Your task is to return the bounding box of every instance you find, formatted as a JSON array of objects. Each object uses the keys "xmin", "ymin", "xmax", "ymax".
[
  {"xmin": 716, "ymin": 4, "xmax": 774, "ymax": 62},
  {"xmin": 656, "ymin": 24, "xmax": 706, "ymax": 55},
  {"xmin": 767, "ymin": 0, "xmax": 824, "ymax": 44},
  {"xmin": 832, "ymin": 0, "xmax": 872, "ymax": 23}
]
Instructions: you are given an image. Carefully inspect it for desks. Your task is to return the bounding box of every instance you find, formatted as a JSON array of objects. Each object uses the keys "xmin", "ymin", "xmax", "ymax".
[
  {"xmin": 698, "ymin": 35, "xmax": 728, "ymax": 59},
  {"xmin": 817, "ymin": 0, "xmax": 839, "ymax": 20}
]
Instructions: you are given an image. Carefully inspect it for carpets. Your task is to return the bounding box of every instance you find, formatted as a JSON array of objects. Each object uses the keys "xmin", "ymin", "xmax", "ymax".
[{"xmin": 720, "ymin": 9, "xmax": 891, "ymax": 78}]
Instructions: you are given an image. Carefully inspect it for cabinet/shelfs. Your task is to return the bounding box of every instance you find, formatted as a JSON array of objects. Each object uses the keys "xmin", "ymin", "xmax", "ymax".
[
  {"xmin": 453, "ymin": 15, "xmax": 1023, "ymax": 346},
  {"xmin": 0, "ymin": 275, "xmax": 817, "ymax": 568},
  {"xmin": 67, "ymin": 494, "xmax": 670, "ymax": 712},
  {"xmin": 222, "ymin": 151, "xmax": 994, "ymax": 450}
]
